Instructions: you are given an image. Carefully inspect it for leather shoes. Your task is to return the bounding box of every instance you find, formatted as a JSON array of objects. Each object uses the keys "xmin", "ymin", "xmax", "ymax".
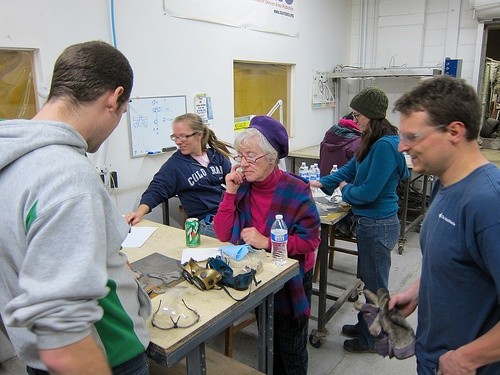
[
  {"xmin": 344, "ymin": 335, "xmax": 370, "ymax": 352},
  {"xmin": 342, "ymin": 324, "xmax": 363, "ymax": 335}
]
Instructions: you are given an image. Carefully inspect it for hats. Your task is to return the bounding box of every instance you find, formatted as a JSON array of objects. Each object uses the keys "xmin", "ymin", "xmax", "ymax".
[
  {"xmin": 350, "ymin": 87, "xmax": 388, "ymax": 119},
  {"xmin": 248, "ymin": 115, "xmax": 288, "ymax": 159}
]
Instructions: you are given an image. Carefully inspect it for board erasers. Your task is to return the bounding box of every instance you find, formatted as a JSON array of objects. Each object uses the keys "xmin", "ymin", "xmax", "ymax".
[{"xmin": 162, "ymin": 146, "xmax": 177, "ymax": 152}]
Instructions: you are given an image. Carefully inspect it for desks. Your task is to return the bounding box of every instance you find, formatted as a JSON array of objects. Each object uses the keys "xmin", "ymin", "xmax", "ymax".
[
  {"xmin": 118, "ymin": 214, "xmax": 300, "ymax": 375},
  {"xmin": 308, "ymin": 196, "xmax": 364, "ymax": 348},
  {"xmin": 287, "ymin": 144, "xmax": 428, "ymax": 255}
]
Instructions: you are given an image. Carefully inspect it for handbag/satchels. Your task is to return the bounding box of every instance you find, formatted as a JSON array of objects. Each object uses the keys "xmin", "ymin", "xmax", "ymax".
[{"xmin": 333, "ymin": 211, "xmax": 357, "ymax": 240}]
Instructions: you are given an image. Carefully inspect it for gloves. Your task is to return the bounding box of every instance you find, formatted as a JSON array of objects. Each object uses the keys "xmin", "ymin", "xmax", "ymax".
[{"xmin": 353, "ymin": 288, "xmax": 417, "ymax": 360}]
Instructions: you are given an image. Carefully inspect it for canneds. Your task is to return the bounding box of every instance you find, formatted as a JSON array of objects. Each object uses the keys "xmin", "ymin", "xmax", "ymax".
[{"xmin": 185, "ymin": 218, "xmax": 201, "ymax": 247}]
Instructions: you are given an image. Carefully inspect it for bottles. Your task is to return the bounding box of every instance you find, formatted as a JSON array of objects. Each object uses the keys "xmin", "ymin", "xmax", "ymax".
[
  {"xmin": 330, "ymin": 165, "xmax": 339, "ymax": 174},
  {"xmin": 271, "ymin": 214, "xmax": 288, "ymax": 266},
  {"xmin": 299, "ymin": 161, "xmax": 320, "ymax": 181}
]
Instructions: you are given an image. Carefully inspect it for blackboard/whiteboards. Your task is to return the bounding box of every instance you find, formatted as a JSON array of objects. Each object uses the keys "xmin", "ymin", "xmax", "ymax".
[{"xmin": 127, "ymin": 95, "xmax": 187, "ymax": 159}]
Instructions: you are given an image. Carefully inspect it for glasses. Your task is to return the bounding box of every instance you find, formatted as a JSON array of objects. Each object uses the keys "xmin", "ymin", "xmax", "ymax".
[
  {"xmin": 181, "ymin": 258, "xmax": 222, "ymax": 291},
  {"xmin": 151, "ymin": 298, "xmax": 200, "ymax": 331},
  {"xmin": 399, "ymin": 122, "xmax": 451, "ymax": 148},
  {"xmin": 351, "ymin": 113, "xmax": 364, "ymax": 120},
  {"xmin": 170, "ymin": 131, "xmax": 200, "ymax": 141},
  {"xmin": 209, "ymin": 258, "xmax": 256, "ymax": 291},
  {"xmin": 234, "ymin": 151, "xmax": 272, "ymax": 164}
]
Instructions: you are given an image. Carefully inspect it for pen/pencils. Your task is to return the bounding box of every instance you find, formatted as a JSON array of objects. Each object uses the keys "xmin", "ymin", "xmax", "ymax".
[{"xmin": 129, "ymin": 223, "xmax": 131, "ymax": 233}]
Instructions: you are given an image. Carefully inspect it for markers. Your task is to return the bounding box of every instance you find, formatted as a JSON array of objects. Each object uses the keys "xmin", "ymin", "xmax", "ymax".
[{"xmin": 148, "ymin": 151, "xmax": 160, "ymax": 154}]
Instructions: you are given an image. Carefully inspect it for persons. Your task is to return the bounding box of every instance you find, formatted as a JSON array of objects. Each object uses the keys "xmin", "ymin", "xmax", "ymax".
[
  {"xmin": 0, "ymin": 40, "xmax": 153, "ymax": 375},
  {"xmin": 388, "ymin": 75, "xmax": 500, "ymax": 375},
  {"xmin": 308, "ymin": 86, "xmax": 410, "ymax": 354},
  {"xmin": 212, "ymin": 116, "xmax": 321, "ymax": 375},
  {"xmin": 318, "ymin": 112, "xmax": 363, "ymax": 177},
  {"xmin": 125, "ymin": 113, "xmax": 234, "ymax": 239}
]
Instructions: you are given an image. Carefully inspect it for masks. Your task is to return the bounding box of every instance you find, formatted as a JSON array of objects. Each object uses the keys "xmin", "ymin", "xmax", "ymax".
[{"xmin": 220, "ymin": 243, "xmax": 255, "ymax": 260}]
